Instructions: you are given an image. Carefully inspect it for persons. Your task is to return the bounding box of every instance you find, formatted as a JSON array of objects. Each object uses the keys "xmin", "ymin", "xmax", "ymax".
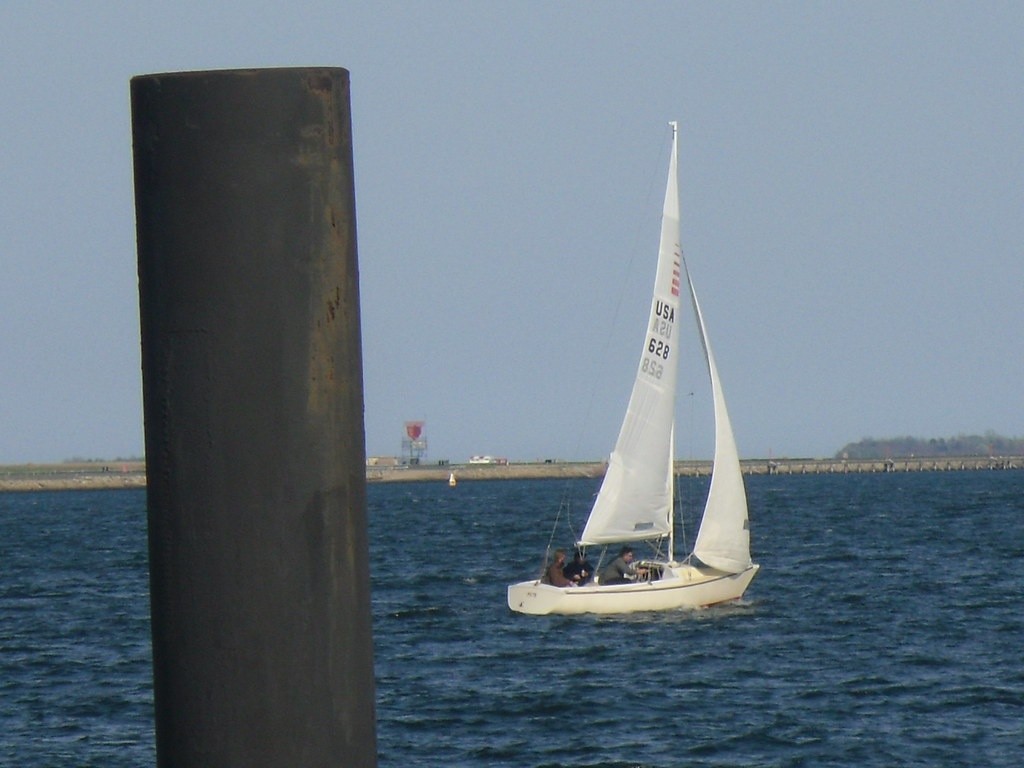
[
  {"xmin": 597, "ymin": 546, "xmax": 646, "ymax": 586},
  {"xmin": 543, "ymin": 550, "xmax": 578, "ymax": 587},
  {"xmin": 563, "ymin": 551, "xmax": 594, "ymax": 587}
]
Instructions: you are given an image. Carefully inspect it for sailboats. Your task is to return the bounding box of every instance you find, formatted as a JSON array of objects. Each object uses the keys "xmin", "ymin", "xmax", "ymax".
[{"xmin": 507, "ymin": 120, "xmax": 761, "ymax": 615}]
